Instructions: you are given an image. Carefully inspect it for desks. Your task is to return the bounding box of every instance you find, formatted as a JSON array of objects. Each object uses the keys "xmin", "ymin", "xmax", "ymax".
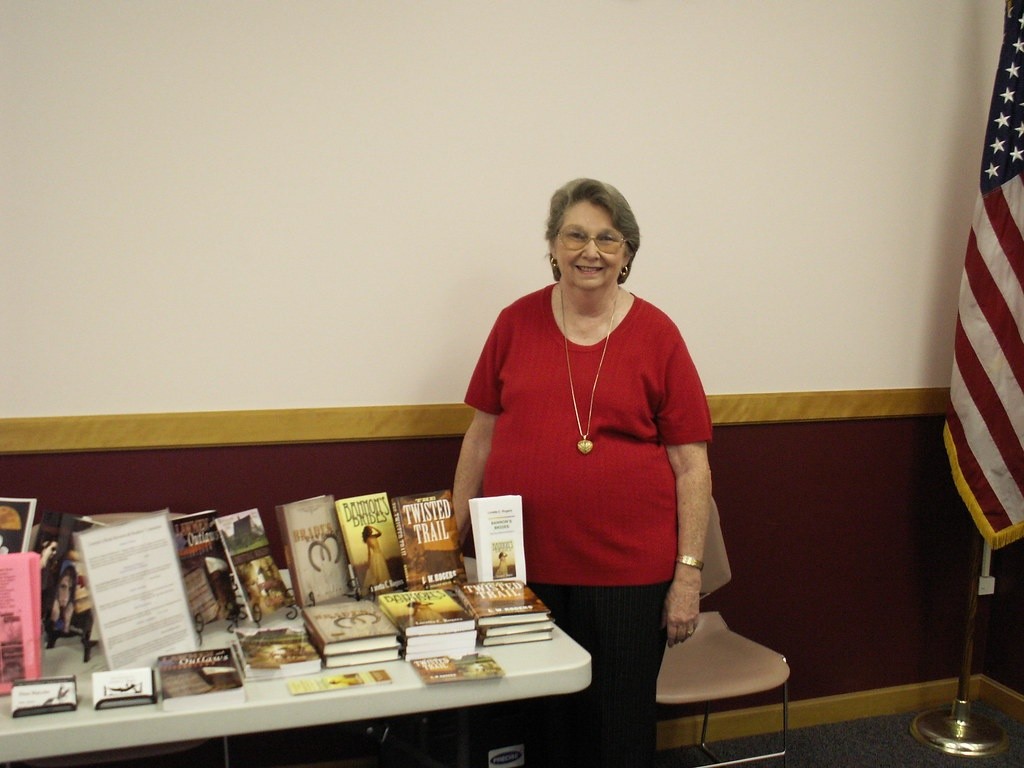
[{"xmin": 0, "ymin": 556, "xmax": 593, "ymax": 768}]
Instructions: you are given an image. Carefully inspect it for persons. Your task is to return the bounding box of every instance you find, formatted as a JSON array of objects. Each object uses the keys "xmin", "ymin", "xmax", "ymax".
[
  {"xmin": 407, "ymin": 602, "xmax": 447, "ymax": 626},
  {"xmin": 495, "ymin": 551, "xmax": 509, "ymax": 578},
  {"xmin": 36, "ymin": 532, "xmax": 78, "ymax": 633},
  {"xmin": 450, "ymin": 179, "xmax": 713, "ymax": 768},
  {"xmin": 361, "ymin": 526, "xmax": 393, "ymax": 596},
  {"xmin": 242, "ymin": 565, "xmax": 287, "ymax": 600}
]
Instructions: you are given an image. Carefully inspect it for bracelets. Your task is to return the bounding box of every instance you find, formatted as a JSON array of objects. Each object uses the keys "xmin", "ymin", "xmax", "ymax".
[{"xmin": 677, "ymin": 553, "xmax": 704, "ymax": 571}]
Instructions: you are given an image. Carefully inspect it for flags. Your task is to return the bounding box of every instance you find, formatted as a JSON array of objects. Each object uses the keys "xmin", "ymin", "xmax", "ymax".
[{"xmin": 943, "ymin": 0, "xmax": 1023, "ymax": 546}]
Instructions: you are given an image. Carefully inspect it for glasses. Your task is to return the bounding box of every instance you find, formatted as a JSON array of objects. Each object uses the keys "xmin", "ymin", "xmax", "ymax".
[{"xmin": 556, "ymin": 224, "xmax": 628, "ymax": 254}]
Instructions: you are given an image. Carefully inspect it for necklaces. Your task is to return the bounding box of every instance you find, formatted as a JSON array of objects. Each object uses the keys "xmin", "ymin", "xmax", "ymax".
[{"xmin": 558, "ymin": 280, "xmax": 618, "ymax": 455}]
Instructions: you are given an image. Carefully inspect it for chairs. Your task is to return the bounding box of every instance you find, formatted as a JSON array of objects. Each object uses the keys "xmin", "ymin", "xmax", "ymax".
[{"xmin": 656, "ymin": 496, "xmax": 790, "ymax": 768}]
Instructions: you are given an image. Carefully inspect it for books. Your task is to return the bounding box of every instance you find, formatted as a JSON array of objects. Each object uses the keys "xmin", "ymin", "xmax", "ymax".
[{"xmin": 0, "ymin": 489, "xmax": 555, "ymax": 718}]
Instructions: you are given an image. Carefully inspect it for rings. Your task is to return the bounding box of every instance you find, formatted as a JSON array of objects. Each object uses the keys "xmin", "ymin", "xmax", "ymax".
[{"xmin": 687, "ymin": 628, "xmax": 693, "ymax": 636}]
